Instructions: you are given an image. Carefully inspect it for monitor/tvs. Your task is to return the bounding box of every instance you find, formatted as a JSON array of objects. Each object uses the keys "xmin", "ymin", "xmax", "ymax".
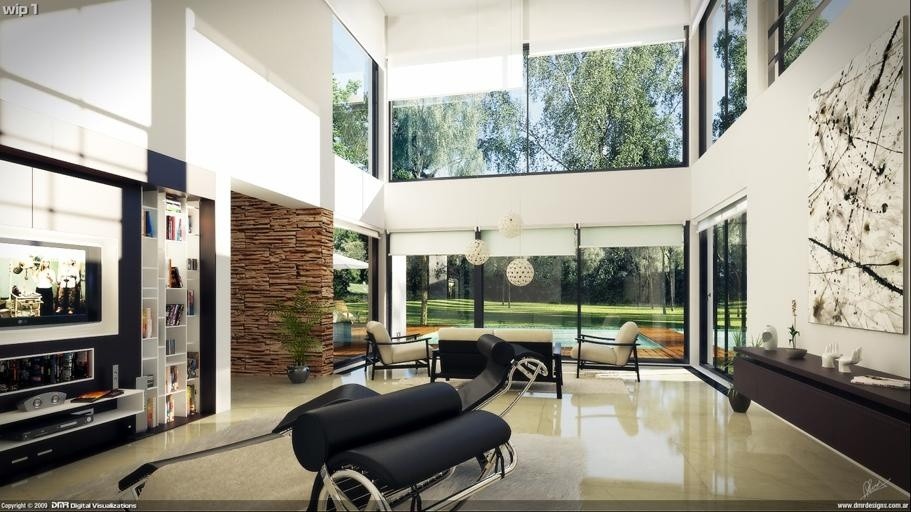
[
  {"xmin": 147, "ymin": 338, "xmax": 200, "ymax": 431},
  {"xmin": 187, "ymin": 289, "xmax": 195, "ymax": 315},
  {"xmin": 187, "ymin": 258, "xmax": 198, "ymax": 270},
  {"xmin": 70, "ymin": 389, "xmax": 124, "ymax": 404},
  {"xmin": 145, "ymin": 198, "xmax": 192, "ymax": 240},
  {"xmin": 166, "ymin": 304, "xmax": 184, "ymax": 326},
  {"xmin": 143, "ymin": 308, "xmax": 153, "ymax": 339}
]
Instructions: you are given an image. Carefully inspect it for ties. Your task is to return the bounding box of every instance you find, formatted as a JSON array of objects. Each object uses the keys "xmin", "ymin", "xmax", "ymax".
[{"xmin": 0, "ymin": 237, "xmax": 102, "ymax": 327}]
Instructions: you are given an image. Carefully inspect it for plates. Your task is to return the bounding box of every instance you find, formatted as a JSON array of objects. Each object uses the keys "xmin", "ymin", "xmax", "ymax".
[
  {"xmin": 140, "ymin": 184, "xmax": 200, "ymax": 436},
  {"xmin": 733, "ymin": 347, "xmax": 910, "ymax": 501}
]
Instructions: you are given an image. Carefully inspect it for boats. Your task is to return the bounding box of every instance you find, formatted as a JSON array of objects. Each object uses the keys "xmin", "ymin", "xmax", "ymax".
[{"xmin": 16, "ymin": 392, "xmax": 67, "ymax": 412}]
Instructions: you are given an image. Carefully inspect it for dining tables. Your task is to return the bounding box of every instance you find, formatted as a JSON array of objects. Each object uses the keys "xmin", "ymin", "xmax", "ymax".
[
  {"xmin": 262, "ymin": 283, "xmax": 340, "ymax": 383},
  {"xmin": 332, "ymin": 269, "xmax": 354, "ymax": 315},
  {"xmin": 721, "ymin": 325, "xmax": 764, "ymax": 414},
  {"xmin": 783, "ymin": 325, "xmax": 807, "ymax": 357}
]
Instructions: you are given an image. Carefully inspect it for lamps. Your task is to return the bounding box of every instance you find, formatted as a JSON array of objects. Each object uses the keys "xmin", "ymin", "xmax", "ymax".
[
  {"xmin": 570, "ymin": 320, "xmax": 642, "ymax": 383},
  {"xmin": 463, "ymin": 44, "xmax": 535, "ymax": 287},
  {"xmin": 364, "ymin": 320, "xmax": 432, "ymax": 380}
]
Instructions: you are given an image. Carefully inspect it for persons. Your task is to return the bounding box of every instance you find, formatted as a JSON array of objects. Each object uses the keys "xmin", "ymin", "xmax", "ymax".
[{"xmin": 36, "ymin": 257, "xmax": 80, "ymax": 314}]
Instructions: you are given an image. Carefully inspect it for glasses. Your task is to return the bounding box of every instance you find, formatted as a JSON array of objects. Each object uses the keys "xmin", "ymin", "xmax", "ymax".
[{"xmin": 463, "ymin": 44, "xmax": 535, "ymax": 287}]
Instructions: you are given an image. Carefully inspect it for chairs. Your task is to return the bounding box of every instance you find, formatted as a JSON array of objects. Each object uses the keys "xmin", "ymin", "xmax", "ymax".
[
  {"xmin": 56, "ymin": 307, "xmax": 74, "ymax": 314},
  {"xmin": 113, "ymin": 332, "xmax": 549, "ymax": 512},
  {"xmin": 570, "ymin": 320, "xmax": 642, "ymax": 383},
  {"xmin": 364, "ymin": 320, "xmax": 432, "ymax": 380}
]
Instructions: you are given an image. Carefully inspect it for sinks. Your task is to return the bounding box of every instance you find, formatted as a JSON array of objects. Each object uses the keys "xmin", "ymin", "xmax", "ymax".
[{"xmin": 429, "ymin": 326, "xmax": 563, "ymax": 399}]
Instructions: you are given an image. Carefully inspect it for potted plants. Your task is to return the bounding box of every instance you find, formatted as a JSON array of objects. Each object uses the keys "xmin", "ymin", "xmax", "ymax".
[
  {"xmin": 783, "ymin": 325, "xmax": 807, "ymax": 357},
  {"xmin": 721, "ymin": 325, "xmax": 764, "ymax": 414},
  {"xmin": 262, "ymin": 283, "xmax": 340, "ymax": 383},
  {"xmin": 332, "ymin": 269, "xmax": 354, "ymax": 315}
]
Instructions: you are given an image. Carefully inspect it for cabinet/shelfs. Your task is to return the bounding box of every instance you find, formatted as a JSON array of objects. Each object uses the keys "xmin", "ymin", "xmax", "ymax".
[
  {"xmin": 733, "ymin": 347, "xmax": 910, "ymax": 501},
  {"xmin": 140, "ymin": 184, "xmax": 200, "ymax": 436},
  {"xmin": 762, "ymin": 325, "xmax": 777, "ymax": 351}
]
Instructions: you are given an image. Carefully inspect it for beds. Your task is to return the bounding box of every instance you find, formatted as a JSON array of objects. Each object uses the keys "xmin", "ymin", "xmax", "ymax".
[{"xmin": 333, "ymin": 254, "xmax": 369, "ymax": 271}]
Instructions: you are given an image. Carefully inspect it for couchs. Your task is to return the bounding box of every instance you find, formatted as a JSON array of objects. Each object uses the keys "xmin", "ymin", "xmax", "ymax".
[{"xmin": 429, "ymin": 326, "xmax": 563, "ymax": 399}]
